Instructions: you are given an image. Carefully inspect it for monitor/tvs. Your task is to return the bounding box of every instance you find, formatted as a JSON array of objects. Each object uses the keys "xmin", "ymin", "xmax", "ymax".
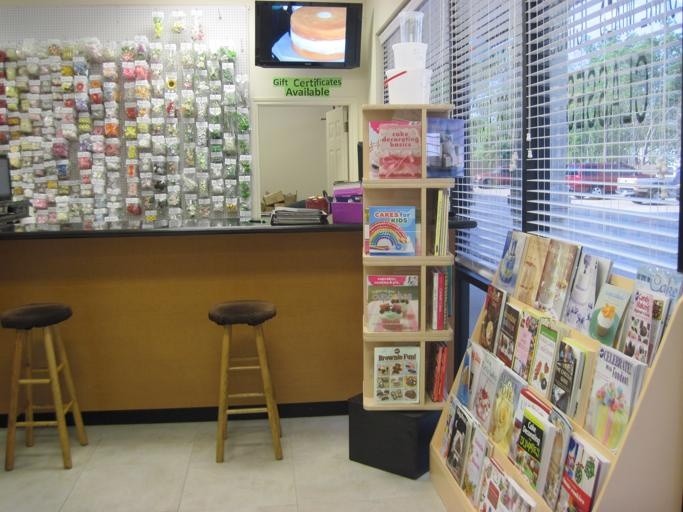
[{"xmin": 254, "ymin": 0, "xmax": 363, "ymax": 70}]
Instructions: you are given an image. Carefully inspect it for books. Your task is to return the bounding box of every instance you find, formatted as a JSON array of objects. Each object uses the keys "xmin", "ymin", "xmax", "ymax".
[
  {"xmin": 427, "ymin": 341, "xmax": 448, "ymax": 402},
  {"xmin": 364, "ymin": 266, "xmax": 451, "ymax": 332},
  {"xmin": 455, "ymin": 338, "xmax": 611, "ymax": 512},
  {"xmin": 364, "ymin": 206, "xmax": 416, "ymax": 256},
  {"xmin": 479, "ymin": 284, "xmax": 507, "ymax": 355},
  {"xmin": 368, "ymin": 121, "xmax": 422, "ymax": 178},
  {"xmin": 495, "ymin": 231, "xmax": 683, "ymax": 367},
  {"xmin": 373, "ymin": 346, "xmax": 420, "ymax": 404},
  {"xmin": 435, "ymin": 395, "xmax": 482, "ymax": 488},
  {"xmin": 511, "ymin": 309, "xmax": 651, "ymax": 456},
  {"xmin": 429, "ymin": 190, "xmax": 449, "ymax": 256}
]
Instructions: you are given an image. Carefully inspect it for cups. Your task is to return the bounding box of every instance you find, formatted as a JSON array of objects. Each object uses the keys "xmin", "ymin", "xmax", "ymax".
[{"xmin": 399, "ymin": 11, "xmax": 424, "ymax": 43}]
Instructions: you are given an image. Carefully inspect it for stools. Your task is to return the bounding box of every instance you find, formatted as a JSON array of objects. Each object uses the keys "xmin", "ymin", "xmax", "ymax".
[
  {"xmin": 206, "ymin": 299, "xmax": 285, "ymax": 463},
  {"xmin": 0, "ymin": 304, "xmax": 88, "ymax": 471}
]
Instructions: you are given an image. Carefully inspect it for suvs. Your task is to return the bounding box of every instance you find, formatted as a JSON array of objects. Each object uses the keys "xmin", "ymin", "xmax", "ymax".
[{"xmin": 566, "ymin": 163, "xmax": 656, "ymax": 198}]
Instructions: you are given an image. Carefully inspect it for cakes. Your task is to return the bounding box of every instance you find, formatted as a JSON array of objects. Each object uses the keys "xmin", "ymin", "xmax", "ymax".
[{"xmin": 290, "ymin": 6, "xmax": 346, "ymax": 60}]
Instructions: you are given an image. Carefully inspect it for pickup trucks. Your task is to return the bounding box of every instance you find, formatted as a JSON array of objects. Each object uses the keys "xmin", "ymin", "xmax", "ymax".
[{"xmin": 617, "ymin": 167, "xmax": 681, "ymax": 205}]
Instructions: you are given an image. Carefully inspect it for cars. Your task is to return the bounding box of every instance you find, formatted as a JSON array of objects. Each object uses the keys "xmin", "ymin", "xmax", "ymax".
[{"xmin": 474, "ymin": 167, "xmax": 512, "ymax": 188}]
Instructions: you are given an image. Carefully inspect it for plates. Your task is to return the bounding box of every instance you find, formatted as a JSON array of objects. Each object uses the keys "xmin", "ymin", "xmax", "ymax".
[{"xmin": 270, "ymin": 31, "xmax": 346, "ymax": 64}]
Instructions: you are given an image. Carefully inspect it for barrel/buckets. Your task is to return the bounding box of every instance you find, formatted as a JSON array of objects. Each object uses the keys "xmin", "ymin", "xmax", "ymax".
[{"xmin": 384, "ymin": 67, "xmax": 432, "ymax": 104}]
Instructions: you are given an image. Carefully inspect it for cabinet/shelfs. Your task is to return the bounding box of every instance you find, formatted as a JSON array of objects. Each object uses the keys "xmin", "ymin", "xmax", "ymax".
[
  {"xmin": 361, "ymin": 103, "xmax": 456, "ymax": 410},
  {"xmin": 429, "ymin": 261, "xmax": 683, "ymax": 512}
]
